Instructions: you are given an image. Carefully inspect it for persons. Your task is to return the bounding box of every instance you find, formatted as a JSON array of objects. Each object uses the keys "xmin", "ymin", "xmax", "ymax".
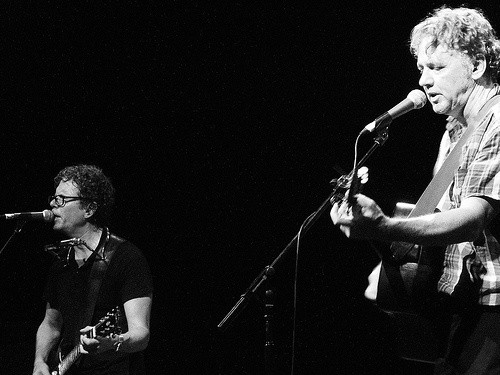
[
  {"xmin": 32, "ymin": 163, "xmax": 152, "ymax": 375},
  {"xmin": 327, "ymin": 4, "xmax": 500, "ymax": 375}
]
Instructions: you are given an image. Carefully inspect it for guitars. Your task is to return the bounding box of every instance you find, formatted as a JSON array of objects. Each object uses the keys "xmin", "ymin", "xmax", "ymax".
[
  {"xmin": 49, "ymin": 305, "xmax": 124, "ymax": 375},
  {"xmin": 329, "ymin": 166, "xmax": 434, "ymax": 320}
]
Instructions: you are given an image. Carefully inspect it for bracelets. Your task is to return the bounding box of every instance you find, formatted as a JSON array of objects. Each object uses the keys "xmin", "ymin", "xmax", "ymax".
[{"xmin": 113, "ymin": 335, "xmax": 124, "ymax": 353}]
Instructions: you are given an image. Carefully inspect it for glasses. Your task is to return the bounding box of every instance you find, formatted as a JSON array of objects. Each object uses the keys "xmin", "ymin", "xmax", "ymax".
[{"xmin": 47, "ymin": 193, "xmax": 91, "ymax": 207}]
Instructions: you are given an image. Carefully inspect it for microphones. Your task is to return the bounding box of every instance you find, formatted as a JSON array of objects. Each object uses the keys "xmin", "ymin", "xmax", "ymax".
[
  {"xmin": 2, "ymin": 209, "xmax": 55, "ymax": 223},
  {"xmin": 362, "ymin": 89, "xmax": 427, "ymax": 134}
]
